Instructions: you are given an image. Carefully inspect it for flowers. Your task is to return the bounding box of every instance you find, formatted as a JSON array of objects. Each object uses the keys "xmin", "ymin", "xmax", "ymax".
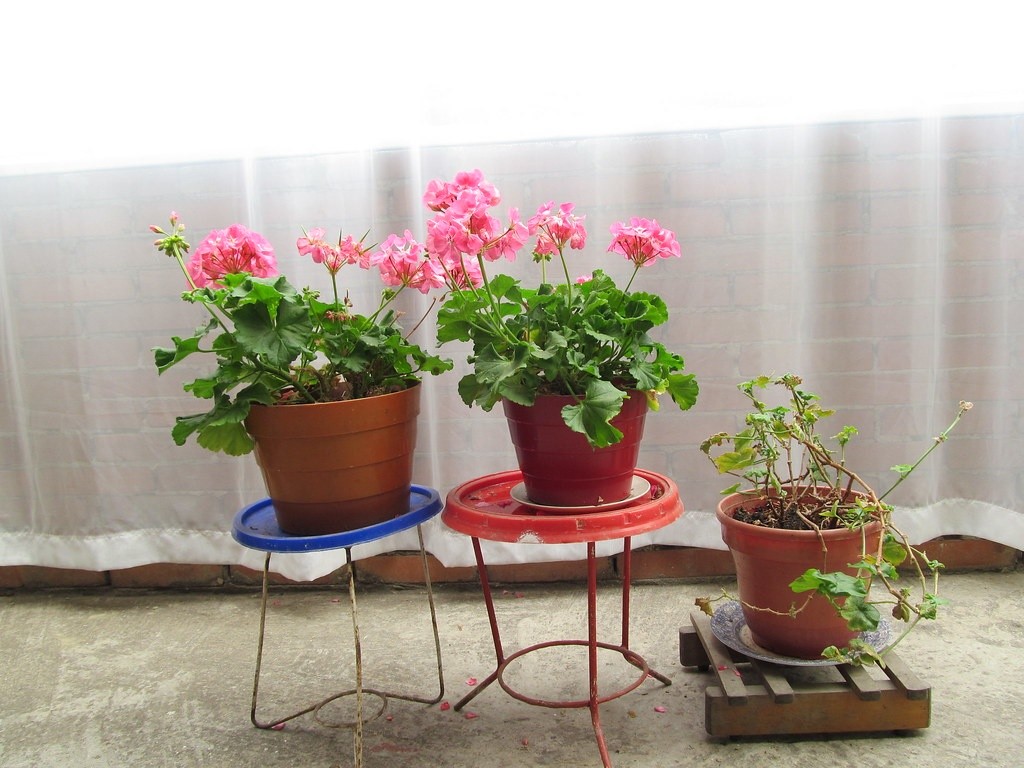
[
  {"xmin": 423, "ymin": 170, "xmax": 702, "ymax": 452},
  {"xmin": 149, "ymin": 213, "xmax": 453, "ymax": 458}
]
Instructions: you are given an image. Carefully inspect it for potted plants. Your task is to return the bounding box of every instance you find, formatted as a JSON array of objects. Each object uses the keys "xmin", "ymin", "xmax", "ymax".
[{"xmin": 695, "ymin": 372, "xmax": 974, "ymax": 667}]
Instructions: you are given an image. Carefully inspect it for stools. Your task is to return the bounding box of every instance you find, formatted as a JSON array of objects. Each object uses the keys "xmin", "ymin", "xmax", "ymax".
[
  {"xmin": 231, "ymin": 483, "xmax": 444, "ymax": 768},
  {"xmin": 441, "ymin": 469, "xmax": 682, "ymax": 768}
]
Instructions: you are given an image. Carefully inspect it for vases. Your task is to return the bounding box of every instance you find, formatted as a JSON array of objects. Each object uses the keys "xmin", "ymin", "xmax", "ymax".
[
  {"xmin": 500, "ymin": 376, "xmax": 648, "ymax": 507},
  {"xmin": 243, "ymin": 379, "xmax": 423, "ymax": 537}
]
